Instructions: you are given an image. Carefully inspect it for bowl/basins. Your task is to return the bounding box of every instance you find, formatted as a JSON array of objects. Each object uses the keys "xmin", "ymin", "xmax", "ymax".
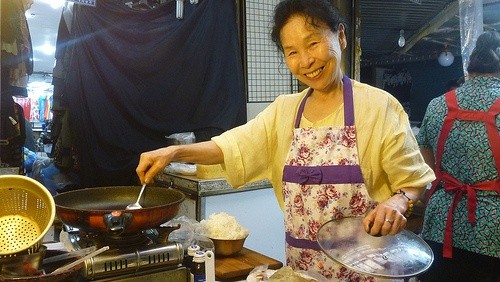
[
  {"xmin": 0, "ymin": 245, "xmax": 47, "ymax": 276},
  {"xmin": 0, "ymin": 249, "xmax": 83, "ymax": 282},
  {"xmin": 206, "ymin": 232, "xmax": 248, "ymax": 255}
]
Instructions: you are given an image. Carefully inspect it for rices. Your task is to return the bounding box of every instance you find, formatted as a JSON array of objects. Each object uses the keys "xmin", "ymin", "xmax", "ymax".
[{"xmin": 199, "ymin": 212, "xmax": 249, "ymax": 240}]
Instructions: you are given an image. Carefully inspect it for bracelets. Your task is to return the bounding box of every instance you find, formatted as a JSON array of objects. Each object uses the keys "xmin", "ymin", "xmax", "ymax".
[{"xmin": 390, "ymin": 188, "xmax": 414, "ymax": 219}]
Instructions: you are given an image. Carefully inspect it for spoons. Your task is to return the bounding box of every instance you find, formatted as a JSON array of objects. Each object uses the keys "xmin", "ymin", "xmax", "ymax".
[{"xmin": 125, "ymin": 184, "xmax": 147, "ymax": 209}]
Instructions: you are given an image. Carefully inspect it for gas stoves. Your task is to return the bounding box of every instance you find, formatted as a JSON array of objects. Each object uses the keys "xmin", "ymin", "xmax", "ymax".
[{"xmin": 59, "ymin": 225, "xmax": 185, "ymax": 280}]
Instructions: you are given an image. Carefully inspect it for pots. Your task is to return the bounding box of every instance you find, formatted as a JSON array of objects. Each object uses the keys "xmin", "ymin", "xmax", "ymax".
[{"xmin": 53, "ymin": 184, "xmax": 186, "ymax": 234}]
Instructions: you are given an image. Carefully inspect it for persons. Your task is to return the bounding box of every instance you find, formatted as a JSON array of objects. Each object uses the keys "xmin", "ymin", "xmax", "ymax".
[
  {"xmin": 415, "ymin": 31, "xmax": 500, "ymax": 282},
  {"xmin": 136, "ymin": 0, "xmax": 436, "ymax": 282}
]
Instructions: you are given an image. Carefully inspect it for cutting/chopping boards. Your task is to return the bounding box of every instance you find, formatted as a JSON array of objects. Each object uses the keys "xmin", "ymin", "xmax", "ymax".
[{"xmin": 215, "ymin": 247, "xmax": 283, "ymax": 282}]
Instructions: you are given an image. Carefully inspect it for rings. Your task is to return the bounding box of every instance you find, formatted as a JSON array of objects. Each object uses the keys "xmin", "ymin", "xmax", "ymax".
[{"xmin": 384, "ymin": 218, "xmax": 394, "ymax": 222}]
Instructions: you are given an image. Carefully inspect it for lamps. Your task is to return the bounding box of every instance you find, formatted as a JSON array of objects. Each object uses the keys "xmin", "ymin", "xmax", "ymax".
[
  {"xmin": 398, "ymin": 29, "xmax": 406, "ymax": 48},
  {"xmin": 438, "ymin": 44, "xmax": 454, "ymax": 67}
]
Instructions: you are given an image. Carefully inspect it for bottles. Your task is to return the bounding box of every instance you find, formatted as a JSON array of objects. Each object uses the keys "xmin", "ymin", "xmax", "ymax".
[
  {"xmin": 186, "ymin": 245, "xmax": 200, "ymax": 282},
  {"xmin": 190, "ymin": 252, "xmax": 206, "ymax": 282}
]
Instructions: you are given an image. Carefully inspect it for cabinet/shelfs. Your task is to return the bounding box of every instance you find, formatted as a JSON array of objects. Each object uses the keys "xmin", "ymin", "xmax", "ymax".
[{"xmin": 158, "ymin": 173, "xmax": 286, "ymax": 266}]
